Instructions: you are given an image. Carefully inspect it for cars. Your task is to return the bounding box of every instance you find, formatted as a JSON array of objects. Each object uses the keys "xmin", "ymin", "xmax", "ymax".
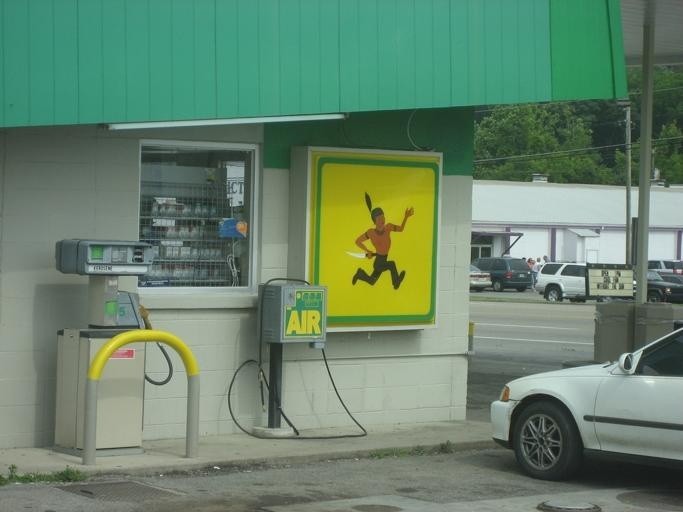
[
  {"xmin": 469, "ymin": 263, "xmax": 492, "ymax": 292},
  {"xmin": 489, "ymin": 325, "xmax": 683, "ymax": 480},
  {"xmin": 648, "ymin": 269, "xmax": 683, "ymax": 302}
]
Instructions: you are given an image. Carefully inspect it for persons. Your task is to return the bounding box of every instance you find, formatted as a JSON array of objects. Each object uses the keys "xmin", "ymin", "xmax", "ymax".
[
  {"xmin": 351, "ymin": 188, "xmax": 415, "ymax": 292},
  {"xmin": 521, "ymin": 255, "xmax": 549, "ymax": 291}
]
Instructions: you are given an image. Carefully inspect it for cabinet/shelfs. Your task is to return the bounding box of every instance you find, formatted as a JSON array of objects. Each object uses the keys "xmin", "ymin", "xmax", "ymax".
[{"xmin": 140, "ymin": 182, "xmax": 236, "ymax": 287}]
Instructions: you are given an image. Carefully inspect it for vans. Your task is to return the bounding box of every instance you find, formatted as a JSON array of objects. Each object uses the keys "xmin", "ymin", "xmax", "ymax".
[
  {"xmin": 472, "ymin": 256, "xmax": 533, "ymax": 292},
  {"xmin": 648, "ymin": 260, "xmax": 682, "ymax": 274},
  {"xmin": 537, "ymin": 262, "xmax": 637, "ymax": 302}
]
